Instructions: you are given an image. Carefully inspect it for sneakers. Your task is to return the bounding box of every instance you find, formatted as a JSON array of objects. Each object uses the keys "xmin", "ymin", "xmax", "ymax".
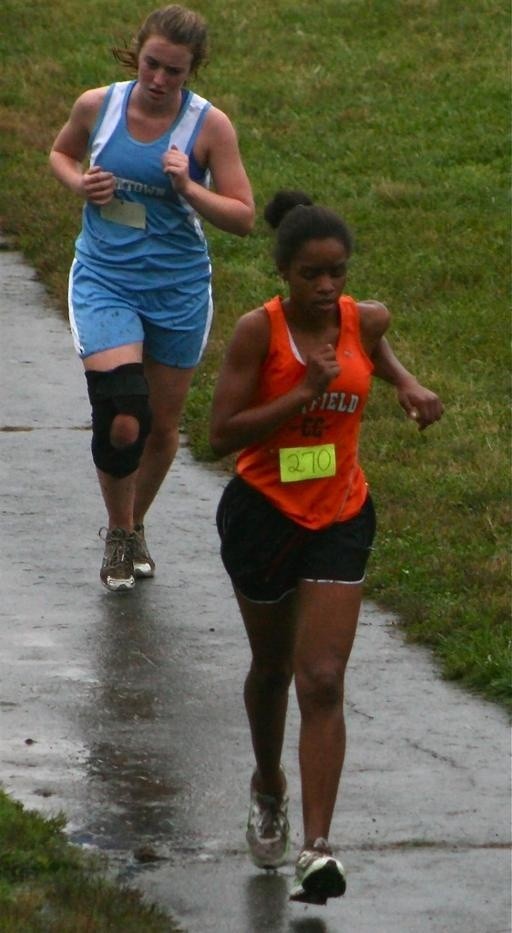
[
  {"xmin": 246, "ymin": 764, "xmax": 289, "ymax": 871},
  {"xmin": 289, "ymin": 837, "xmax": 347, "ymax": 904},
  {"xmin": 99, "ymin": 525, "xmax": 155, "ymax": 591}
]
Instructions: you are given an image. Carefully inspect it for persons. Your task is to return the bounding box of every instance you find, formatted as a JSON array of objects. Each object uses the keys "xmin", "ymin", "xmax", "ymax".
[
  {"xmin": 209, "ymin": 191, "xmax": 444, "ymax": 905},
  {"xmin": 49, "ymin": 5, "xmax": 255, "ymax": 592}
]
